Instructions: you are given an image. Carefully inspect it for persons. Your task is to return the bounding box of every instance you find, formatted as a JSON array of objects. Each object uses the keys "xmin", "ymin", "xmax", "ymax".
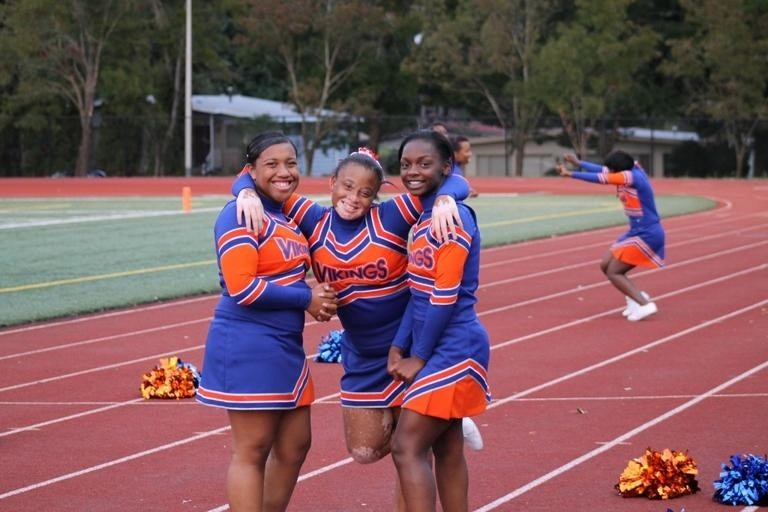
[
  {"xmin": 428, "ymin": 123, "xmax": 450, "ymax": 142},
  {"xmin": 554, "ymin": 150, "xmax": 665, "ymax": 322},
  {"xmin": 386, "ymin": 130, "xmax": 491, "ymax": 512},
  {"xmin": 195, "ymin": 132, "xmax": 339, "ymax": 512},
  {"xmin": 452, "ymin": 137, "xmax": 479, "ymax": 199},
  {"xmin": 230, "ymin": 147, "xmax": 471, "ymax": 512}
]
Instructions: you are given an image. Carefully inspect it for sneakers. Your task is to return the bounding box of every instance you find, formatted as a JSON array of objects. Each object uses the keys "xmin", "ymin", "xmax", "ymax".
[
  {"xmin": 461, "ymin": 418, "xmax": 484, "ymax": 451},
  {"xmin": 622, "ymin": 291, "xmax": 650, "ymax": 317},
  {"xmin": 627, "ymin": 302, "xmax": 657, "ymax": 321}
]
